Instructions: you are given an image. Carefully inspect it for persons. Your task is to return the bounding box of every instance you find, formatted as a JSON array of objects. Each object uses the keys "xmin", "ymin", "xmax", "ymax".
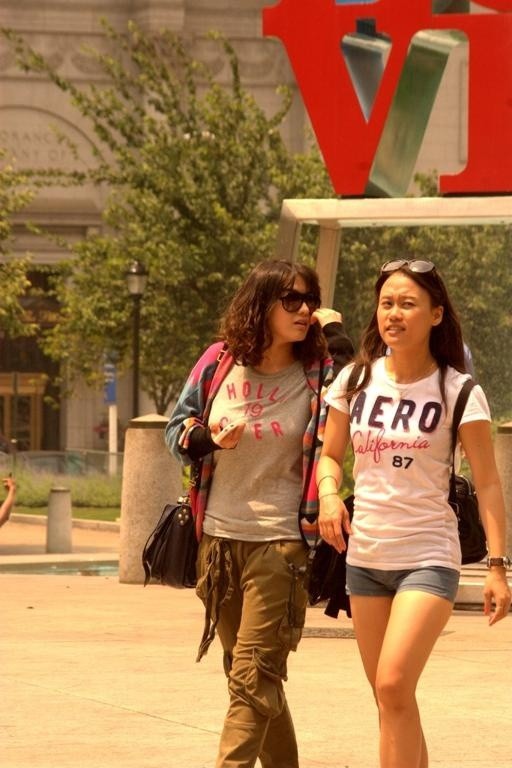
[
  {"xmin": 163, "ymin": 257, "xmax": 356, "ymax": 768},
  {"xmin": 317, "ymin": 259, "xmax": 512, "ymax": 767},
  {"xmin": 0, "ymin": 471, "xmax": 20, "ymax": 528}
]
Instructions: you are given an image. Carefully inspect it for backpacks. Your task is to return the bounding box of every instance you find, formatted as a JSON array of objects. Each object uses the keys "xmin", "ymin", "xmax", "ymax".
[{"xmin": 346, "ymin": 360, "xmax": 488, "ymax": 566}]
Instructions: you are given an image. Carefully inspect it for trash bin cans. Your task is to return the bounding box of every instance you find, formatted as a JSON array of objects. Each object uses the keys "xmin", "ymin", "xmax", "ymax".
[{"xmin": 65, "ymin": 448, "xmax": 83, "ymax": 477}]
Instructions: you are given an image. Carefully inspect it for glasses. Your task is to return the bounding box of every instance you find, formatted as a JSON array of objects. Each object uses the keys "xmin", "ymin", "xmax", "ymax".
[
  {"xmin": 379, "ymin": 257, "xmax": 436, "ymax": 277},
  {"xmin": 281, "ymin": 291, "xmax": 319, "ymax": 312}
]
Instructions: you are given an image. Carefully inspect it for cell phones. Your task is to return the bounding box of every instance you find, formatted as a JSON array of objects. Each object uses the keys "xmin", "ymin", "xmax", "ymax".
[{"xmin": 213, "ymin": 417, "xmax": 245, "ymax": 444}]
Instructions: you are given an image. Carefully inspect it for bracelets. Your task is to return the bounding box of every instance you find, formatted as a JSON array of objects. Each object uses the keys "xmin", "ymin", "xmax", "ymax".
[
  {"xmin": 316, "ymin": 475, "xmax": 338, "ymax": 488},
  {"xmin": 318, "ymin": 490, "xmax": 338, "ymax": 496},
  {"xmin": 485, "ymin": 555, "xmax": 511, "ymax": 570}
]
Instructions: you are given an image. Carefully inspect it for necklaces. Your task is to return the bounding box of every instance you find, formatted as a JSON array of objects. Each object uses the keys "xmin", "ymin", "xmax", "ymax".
[{"xmin": 387, "ymin": 358, "xmax": 438, "ymax": 403}]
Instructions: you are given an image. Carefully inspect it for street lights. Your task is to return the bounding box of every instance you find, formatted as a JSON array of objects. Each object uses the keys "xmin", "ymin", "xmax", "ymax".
[{"xmin": 124, "ymin": 258, "xmax": 148, "ymax": 418}]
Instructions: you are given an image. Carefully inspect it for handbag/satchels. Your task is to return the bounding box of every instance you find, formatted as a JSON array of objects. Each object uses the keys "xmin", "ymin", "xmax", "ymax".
[
  {"xmin": 307, "ymin": 493, "xmax": 355, "ymax": 619},
  {"xmin": 142, "ymin": 494, "xmax": 199, "ymax": 590}
]
